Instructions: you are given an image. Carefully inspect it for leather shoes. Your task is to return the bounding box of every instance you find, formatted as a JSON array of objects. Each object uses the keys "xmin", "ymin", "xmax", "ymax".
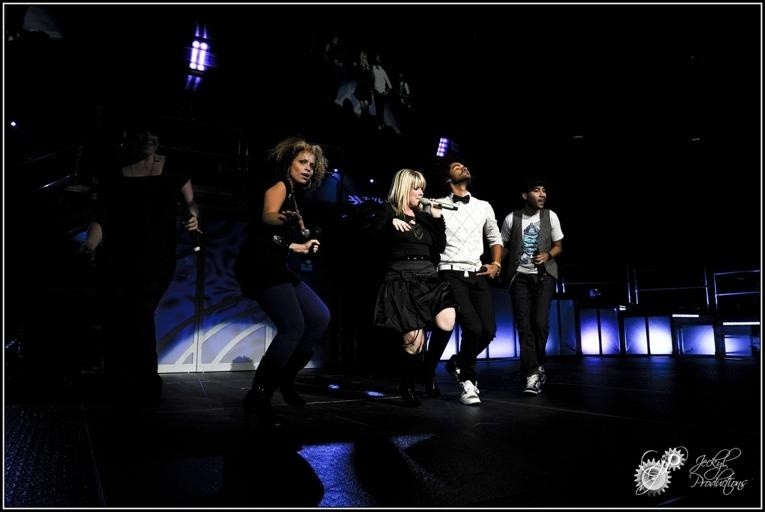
[{"xmin": 400, "ymin": 379, "xmax": 440, "ymax": 408}]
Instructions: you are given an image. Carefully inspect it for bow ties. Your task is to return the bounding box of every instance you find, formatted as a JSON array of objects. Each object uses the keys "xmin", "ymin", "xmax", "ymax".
[{"xmin": 453, "ymin": 195, "xmax": 469, "ymax": 203}]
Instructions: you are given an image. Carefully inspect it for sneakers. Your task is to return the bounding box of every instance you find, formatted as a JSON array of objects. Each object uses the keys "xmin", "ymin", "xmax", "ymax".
[
  {"xmin": 457, "ymin": 380, "xmax": 481, "ymax": 404},
  {"xmin": 523, "ymin": 365, "xmax": 547, "ymax": 394}
]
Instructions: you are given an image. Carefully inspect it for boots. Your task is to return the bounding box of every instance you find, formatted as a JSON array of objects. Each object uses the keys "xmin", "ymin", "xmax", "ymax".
[{"xmin": 244, "ymin": 356, "xmax": 305, "ymax": 432}]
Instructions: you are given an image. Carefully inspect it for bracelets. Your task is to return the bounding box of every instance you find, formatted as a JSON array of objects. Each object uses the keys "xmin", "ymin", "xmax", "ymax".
[
  {"xmin": 492, "ymin": 261, "xmax": 501, "ymax": 270},
  {"xmin": 547, "ymin": 251, "xmax": 552, "ymax": 260}
]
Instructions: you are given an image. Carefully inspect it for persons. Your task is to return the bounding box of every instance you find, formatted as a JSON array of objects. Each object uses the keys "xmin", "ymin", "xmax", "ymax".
[
  {"xmin": 362, "ymin": 168, "xmax": 457, "ymax": 404},
  {"xmin": 233, "ymin": 138, "xmax": 332, "ymax": 434},
  {"xmin": 79, "ymin": 118, "xmax": 202, "ymax": 397},
  {"xmin": 500, "ymin": 186, "xmax": 565, "ymax": 398},
  {"xmin": 427, "ymin": 160, "xmax": 504, "ymax": 405}
]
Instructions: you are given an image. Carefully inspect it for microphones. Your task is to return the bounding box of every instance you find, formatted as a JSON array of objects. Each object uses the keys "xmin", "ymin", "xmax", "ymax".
[
  {"xmin": 534, "ymin": 250, "xmax": 544, "ymax": 281},
  {"xmin": 481, "ymin": 266, "xmax": 487, "ymax": 272},
  {"xmin": 421, "ymin": 198, "xmax": 458, "ymax": 210},
  {"xmin": 182, "ymin": 210, "xmax": 200, "ymax": 253},
  {"xmin": 305, "ymin": 226, "xmax": 322, "ymax": 266}
]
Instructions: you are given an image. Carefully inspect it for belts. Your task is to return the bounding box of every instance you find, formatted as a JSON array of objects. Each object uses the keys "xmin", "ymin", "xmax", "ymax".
[{"xmin": 437, "ymin": 265, "xmax": 480, "ymax": 272}]
